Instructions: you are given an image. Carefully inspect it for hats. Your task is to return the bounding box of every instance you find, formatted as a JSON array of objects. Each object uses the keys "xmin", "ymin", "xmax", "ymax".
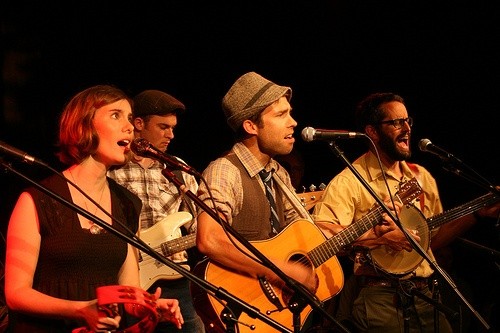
[
  {"xmin": 221, "ymin": 71, "xmax": 292, "ymax": 131},
  {"xmin": 133, "ymin": 90, "xmax": 185, "ymax": 114}
]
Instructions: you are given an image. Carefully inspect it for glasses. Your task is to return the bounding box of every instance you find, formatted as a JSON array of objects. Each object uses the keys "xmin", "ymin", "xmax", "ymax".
[{"xmin": 380, "ymin": 117, "xmax": 413, "ymax": 130}]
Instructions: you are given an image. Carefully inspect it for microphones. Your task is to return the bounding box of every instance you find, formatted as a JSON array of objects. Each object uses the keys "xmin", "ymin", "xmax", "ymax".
[
  {"xmin": 129, "ymin": 137, "xmax": 201, "ymax": 177},
  {"xmin": 0, "ymin": 141, "xmax": 48, "ymax": 168},
  {"xmin": 301, "ymin": 127, "xmax": 366, "ymax": 142},
  {"xmin": 417, "ymin": 138, "xmax": 464, "ymax": 164},
  {"xmin": 343, "ymin": 245, "xmax": 373, "ymax": 253}
]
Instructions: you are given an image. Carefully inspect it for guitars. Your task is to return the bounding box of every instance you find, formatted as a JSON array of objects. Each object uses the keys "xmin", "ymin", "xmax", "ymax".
[
  {"xmin": 118, "ymin": 210, "xmax": 197, "ymax": 290},
  {"xmin": 350, "ymin": 185, "xmax": 500, "ymax": 283},
  {"xmin": 189, "ymin": 177, "xmax": 426, "ymax": 333},
  {"xmin": 289, "ymin": 181, "xmax": 328, "ymax": 213}
]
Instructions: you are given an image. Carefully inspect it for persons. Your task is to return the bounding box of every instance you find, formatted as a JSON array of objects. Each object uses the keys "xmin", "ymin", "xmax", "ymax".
[
  {"xmin": 104, "ymin": 90, "xmax": 205, "ymax": 333},
  {"xmin": 310, "ymin": 93, "xmax": 500, "ymax": 333},
  {"xmin": 4, "ymin": 85, "xmax": 185, "ymax": 332},
  {"xmin": 194, "ymin": 72, "xmax": 402, "ymax": 333}
]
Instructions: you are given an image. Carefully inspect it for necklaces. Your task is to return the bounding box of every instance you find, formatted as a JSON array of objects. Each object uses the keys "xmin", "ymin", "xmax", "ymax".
[{"xmin": 68, "ymin": 168, "xmax": 107, "ymax": 235}]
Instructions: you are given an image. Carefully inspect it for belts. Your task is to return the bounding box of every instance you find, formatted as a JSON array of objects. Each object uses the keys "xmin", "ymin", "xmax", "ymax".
[{"xmin": 367, "ymin": 279, "xmax": 438, "ymax": 289}]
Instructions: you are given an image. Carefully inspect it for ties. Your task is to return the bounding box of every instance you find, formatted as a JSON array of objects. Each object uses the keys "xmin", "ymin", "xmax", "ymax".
[{"xmin": 259, "ymin": 169, "xmax": 280, "ymax": 237}]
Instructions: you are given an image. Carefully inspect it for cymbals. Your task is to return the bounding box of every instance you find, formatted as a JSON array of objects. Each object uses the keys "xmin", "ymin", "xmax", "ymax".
[{"xmin": 70, "ymin": 286, "xmax": 161, "ymax": 333}]
